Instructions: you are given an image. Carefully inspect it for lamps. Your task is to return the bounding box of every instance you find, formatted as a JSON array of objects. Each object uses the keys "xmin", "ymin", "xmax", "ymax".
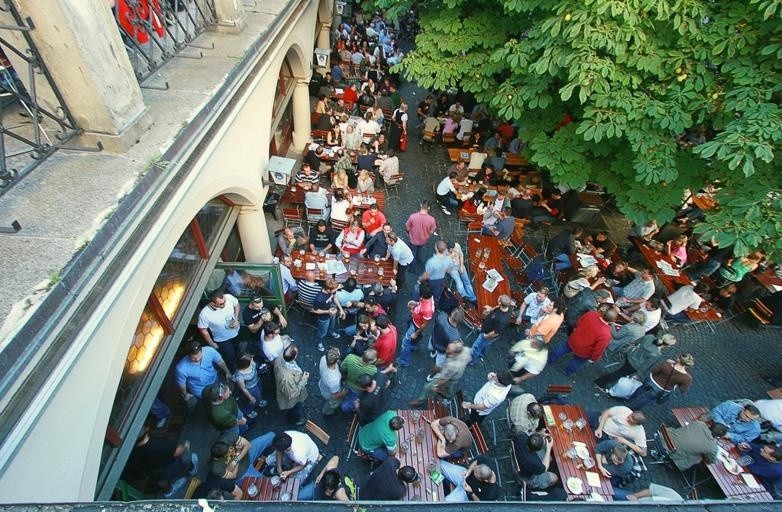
[
  {"xmin": 262, "ymin": 154, "xmax": 298, "ymax": 189},
  {"xmin": 309, "ymin": 47, "xmax": 331, "ymax": 71},
  {"xmin": 335, "ymin": 1, "xmax": 348, "ymax": 17}
]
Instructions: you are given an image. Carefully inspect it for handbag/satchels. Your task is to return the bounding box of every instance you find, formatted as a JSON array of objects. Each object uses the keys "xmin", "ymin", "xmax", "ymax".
[
  {"xmin": 656, "ymin": 391, "xmax": 671, "ymax": 405},
  {"xmin": 610, "ymin": 376, "xmax": 644, "ymax": 399}
]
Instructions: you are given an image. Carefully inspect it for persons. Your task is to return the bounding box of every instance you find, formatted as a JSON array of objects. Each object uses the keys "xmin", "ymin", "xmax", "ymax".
[{"xmin": 120, "ymin": 5, "xmax": 781, "ymax": 503}]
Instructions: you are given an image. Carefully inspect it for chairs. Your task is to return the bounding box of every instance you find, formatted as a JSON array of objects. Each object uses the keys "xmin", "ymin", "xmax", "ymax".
[
  {"xmin": 415, "ymin": 96, "xmax": 782, "ymax": 337},
  {"xmin": 273, "ymin": 61, "xmax": 405, "ymax": 331},
  {"xmin": 235, "ymin": 384, "xmax": 781, "ymax": 503}
]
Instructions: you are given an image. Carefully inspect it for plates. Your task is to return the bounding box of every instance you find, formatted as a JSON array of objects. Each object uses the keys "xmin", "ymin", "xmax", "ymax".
[
  {"xmin": 576, "ymin": 444, "xmax": 588, "ymax": 459},
  {"xmin": 724, "ymin": 461, "xmax": 739, "ymax": 475},
  {"xmin": 567, "ymin": 477, "xmax": 582, "ymax": 495}
]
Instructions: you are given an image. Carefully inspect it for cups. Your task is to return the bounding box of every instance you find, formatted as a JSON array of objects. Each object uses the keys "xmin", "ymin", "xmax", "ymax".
[
  {"xmin": 280, "ymin": 491, "xmax": 292, "ymax": 500},
  {"xmin": 561, "ymin": 449, "xmax": 595, "ymax": 470},
  {"xmin": 558, "ymin": 410, "xmax": 587, "ymax": 432},
  {"xmin": 737, "ymin": 454, "xmax": 754, "ymax": 467},
  {"xmin": 473, "ymin": 246, "xmax": 490, "ymax": 272},
  {"xmin": 295, "ymin": 249, "xmax": 383, "ymax": 280},
  {"xmin": 401, "ymin": 410, "xmax": 424, "ymax": 452},
  {"xmin": 248, "ymin": 485, "xmax": 258, "ymax": 497},
  {"xmin": 271, "ymin": 475, "xmax": 281, "ymax": 487}
]
